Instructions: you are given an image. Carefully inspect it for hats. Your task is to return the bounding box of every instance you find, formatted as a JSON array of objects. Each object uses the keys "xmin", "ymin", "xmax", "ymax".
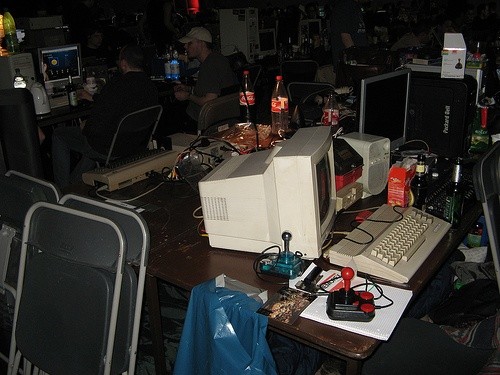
[{"xmin": 178, "ymin": 27, "xmax": 212, "ymax": 44}]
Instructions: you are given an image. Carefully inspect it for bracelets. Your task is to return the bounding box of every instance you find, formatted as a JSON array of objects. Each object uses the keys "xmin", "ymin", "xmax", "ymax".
[{"xmin": 188, "ymin": 93, "xmax": 192, "ymax": 101}]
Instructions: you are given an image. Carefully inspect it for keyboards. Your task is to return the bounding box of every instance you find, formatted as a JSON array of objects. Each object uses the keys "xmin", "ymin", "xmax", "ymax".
[
  {"xmin": 49, "ymin": 96, "xmax": 69, "ymax": 109},
  {"xmin": 82, "ymin": 145, "xmax": 185, "ymax": 192},
  {"xmin": 328, "ymin": 175, "xmax": 475, "ymax": 285}
]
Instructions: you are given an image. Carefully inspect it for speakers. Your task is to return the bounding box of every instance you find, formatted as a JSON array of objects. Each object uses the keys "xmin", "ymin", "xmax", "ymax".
[{"xmin": 335, "ymin": 132, "xmax": 390, "ymax": 199}]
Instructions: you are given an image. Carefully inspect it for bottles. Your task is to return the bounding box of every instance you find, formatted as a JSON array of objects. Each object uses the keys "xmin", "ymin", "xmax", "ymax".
[
  {"xmin": 238, "ymin": 69, "xmax": 256, "ymax": 124},
  {"xmin": 277, "ymin": 32, "xmax": 312, "ymax": 65},
  {"xmin": 14, "ymin": 68, "xmax": 27, "ymax": 88},
  {"xmin": 471, "ymin": 99, "xmax": 490, "ymax": 154},
  {"xmin": 164, "ymin": 49, "xmax": 180, "ymax": 81},
  {"xmin": 82, "ymin": 66, "xmax": 88, "ymax": 84},
  {"xmin": 323, "ymin": 90, "xmax": 341, "ymax": 143},
  {"xmin": 407, "ymin": 155, "xmax": 428, "ymax": 212},
  {"xmin": 442, "ymin": 158, "xmax": 466, "ymax": 229},
  {"xmin": 270, "ymin": 75, "xmax": 289, "ymax": 136},
  {"xmin": 0, "ymin": 8, "xmax": 18, "ymax": 57},
  {"xmin": 467, "ymin": 221, "xmax": 484, "ymax": 248},
  {"xmin": 67, "ymin": 74, "xmax": 78, "ymax": 107}
]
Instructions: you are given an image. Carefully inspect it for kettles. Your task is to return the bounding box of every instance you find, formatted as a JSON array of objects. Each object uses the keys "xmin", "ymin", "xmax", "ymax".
[{"xmin": 30, "ymin": 82, "xmax": 51, "ymax": 115}]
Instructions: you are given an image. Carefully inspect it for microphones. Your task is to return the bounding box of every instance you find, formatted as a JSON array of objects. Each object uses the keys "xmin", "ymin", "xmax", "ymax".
[{"xmin": 235, "ymin": 73, "xmax": 258, "ymax": 151}]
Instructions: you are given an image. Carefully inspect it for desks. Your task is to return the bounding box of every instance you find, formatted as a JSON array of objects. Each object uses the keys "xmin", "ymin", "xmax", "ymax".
[
  {"xmin": 31, "ymin": 82, "xmax": 196, "ymax": 126},
  {"xmin": 9, "ymin": 129, "xmax": 483, "ymax": 375}
]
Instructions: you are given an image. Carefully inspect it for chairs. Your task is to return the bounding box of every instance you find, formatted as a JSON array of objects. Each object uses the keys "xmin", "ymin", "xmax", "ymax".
[{"xmin": 0, "ymin": 55, "xmax": 500, "ymax": 375}]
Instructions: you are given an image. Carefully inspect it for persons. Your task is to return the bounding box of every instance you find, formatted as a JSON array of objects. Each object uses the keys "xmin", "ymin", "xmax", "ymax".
[
  {"xmin": 304, "ymin": 61, "xmax": 375, "ymax": 112},
  {"xmin": 259, "ymin": 2, "xmax": 425, "ymax": 36},
  {"xmin": 324, "ymin": 32, "xmax": 363, "ymax": 66},
  {"xmin": 51, "ymin": 0, "xmax": 96, "ymax": 34},
  {"xmin": 154, "ymin": 2, "xmax": 181, "ymax": 44},
  {"xmin": 151, "ymin": 27, "xmax": 232, "ymax": 151},
  {"xmin": 51, "ymin": 46, "xmax": 158, "ymax": 203},
  {"xmin": 429, "ymin": 0, "xmax": 500, "ymax": 50},
  {"xmin": 391, "ymin": 19, "xmax": 421, "ymax": 51},
  {"xmin": 81, "ymin": 23, "xmax": 111, "ymax": 58}
]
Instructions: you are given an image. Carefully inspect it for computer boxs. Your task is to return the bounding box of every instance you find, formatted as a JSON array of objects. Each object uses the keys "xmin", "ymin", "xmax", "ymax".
[
  {"xmin": 400, "ymin": 71, "xmax": 477, "ymax": 162},
  {"xmin": 220, "ymin": 7, "xmax": 260, "ymax": 64},
  {"xmin": 0, "ymin": 52, "xmax": 37, "ymax": 91}
]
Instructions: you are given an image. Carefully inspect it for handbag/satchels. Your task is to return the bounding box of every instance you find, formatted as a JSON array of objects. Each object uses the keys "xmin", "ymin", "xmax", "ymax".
[{"xmin": 424, "ymin": 261, "xmax": 499, "ymax": 324}]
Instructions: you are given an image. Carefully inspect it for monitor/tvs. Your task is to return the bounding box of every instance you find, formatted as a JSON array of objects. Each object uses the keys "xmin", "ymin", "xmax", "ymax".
[
  {"xmin": 199, "ymin": 125, "xmax": 338, "ymax": 261},
  {"xmin": 38, "ymin": 44, "xmax": 83, "ymax": 83},
  {"xmin": 358, "ymin": 68, "xmax": 413, "ymax": 150},
  {"xmin": 259, "ymin": 29, "xmax": 277, "ymax": 57},
  {"xmin": 308, "ymin": 19, "xmax": 322, "ymax": 40}
]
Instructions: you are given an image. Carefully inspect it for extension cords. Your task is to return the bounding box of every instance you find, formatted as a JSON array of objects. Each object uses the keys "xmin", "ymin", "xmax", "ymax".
[{"xmin": 190, "ymin": 141, "xmax": 226, "ymax": 170}]
